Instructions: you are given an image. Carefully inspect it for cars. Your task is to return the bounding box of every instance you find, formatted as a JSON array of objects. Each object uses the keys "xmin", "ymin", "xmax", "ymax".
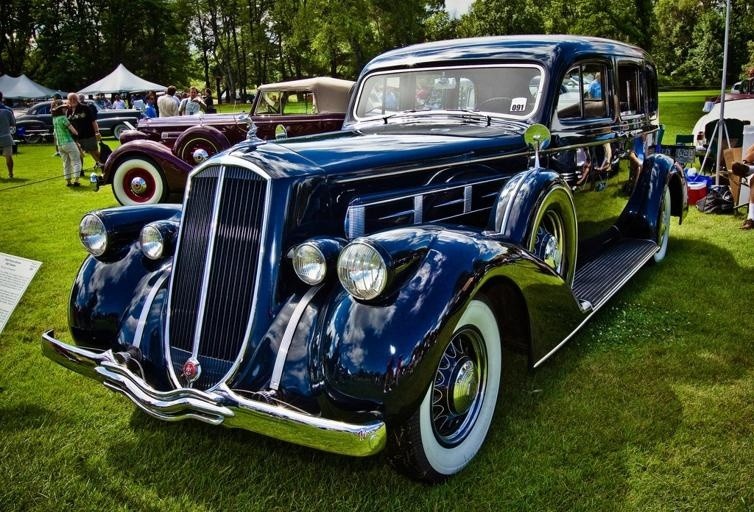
[
  {"xmin": 92, "ymin": 75, "xmax": 359, "ymax": 209},
  {"xmin": 42, "ymin": 33, "xmax": 690, "ymax": 483},
  {"xmin": 9, "ymin": 99, "xmax": 144, "ymax": 144}
]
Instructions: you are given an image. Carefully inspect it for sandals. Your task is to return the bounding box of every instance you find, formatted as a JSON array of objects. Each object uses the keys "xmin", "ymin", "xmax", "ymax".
[{"xmin": 67, "ymin": 182, "xmax": 80, "ymax": 186}]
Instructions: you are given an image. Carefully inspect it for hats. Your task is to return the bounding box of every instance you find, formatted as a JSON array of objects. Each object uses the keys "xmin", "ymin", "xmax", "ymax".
[{"xmin": 50, "ymin": 99, "xmax": 69, "ymax": 111}]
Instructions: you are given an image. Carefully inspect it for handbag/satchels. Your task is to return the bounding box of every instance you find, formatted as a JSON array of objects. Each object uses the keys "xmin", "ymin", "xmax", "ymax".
[{"xmin": 98, "ymin": 140, "xmax": 113, "ymax": 164}]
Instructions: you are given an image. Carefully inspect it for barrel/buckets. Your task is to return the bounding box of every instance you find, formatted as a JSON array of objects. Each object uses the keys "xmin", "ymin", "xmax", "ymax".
[{"xmin": 686, "ymin": 179, "xmax": 708, "ymax": 205}]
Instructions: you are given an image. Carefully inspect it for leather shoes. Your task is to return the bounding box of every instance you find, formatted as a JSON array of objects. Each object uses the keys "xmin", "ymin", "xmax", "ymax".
[{"xmin": 738, "ymin": 219, "xmax": 754, "ymax": 229}]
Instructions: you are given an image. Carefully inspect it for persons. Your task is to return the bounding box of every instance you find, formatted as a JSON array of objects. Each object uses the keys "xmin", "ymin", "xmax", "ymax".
[
  {"xmin": 588, "ymin": 73, "xmax": 604, "ymax": 99},
  {"xmin": 573, "ymin": 144, "xmax": 612, "ymax": 186},
  {"xmin": 732, "ymin": 144, "xmax": 753, "ymax": 230},
  {"xmin": 629, "ymin": 131, "xmax": 644, "ymax": 184},
  {"xmin": 0, "ymin": 90, "xmax": 16, "ymax": 178},
  {"xmin": 50, "ymin": 84, "xmax": 214, "ymax": 187}
]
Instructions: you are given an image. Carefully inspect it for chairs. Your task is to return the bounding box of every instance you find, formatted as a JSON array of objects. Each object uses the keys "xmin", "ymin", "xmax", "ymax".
[
  {"xmin": 695, "ymin": 117, "xmax": 744, "ymax": 173},
  {"xmin": 580, "ymin": 97, "xmax": 629, "ymax": 121}
]
